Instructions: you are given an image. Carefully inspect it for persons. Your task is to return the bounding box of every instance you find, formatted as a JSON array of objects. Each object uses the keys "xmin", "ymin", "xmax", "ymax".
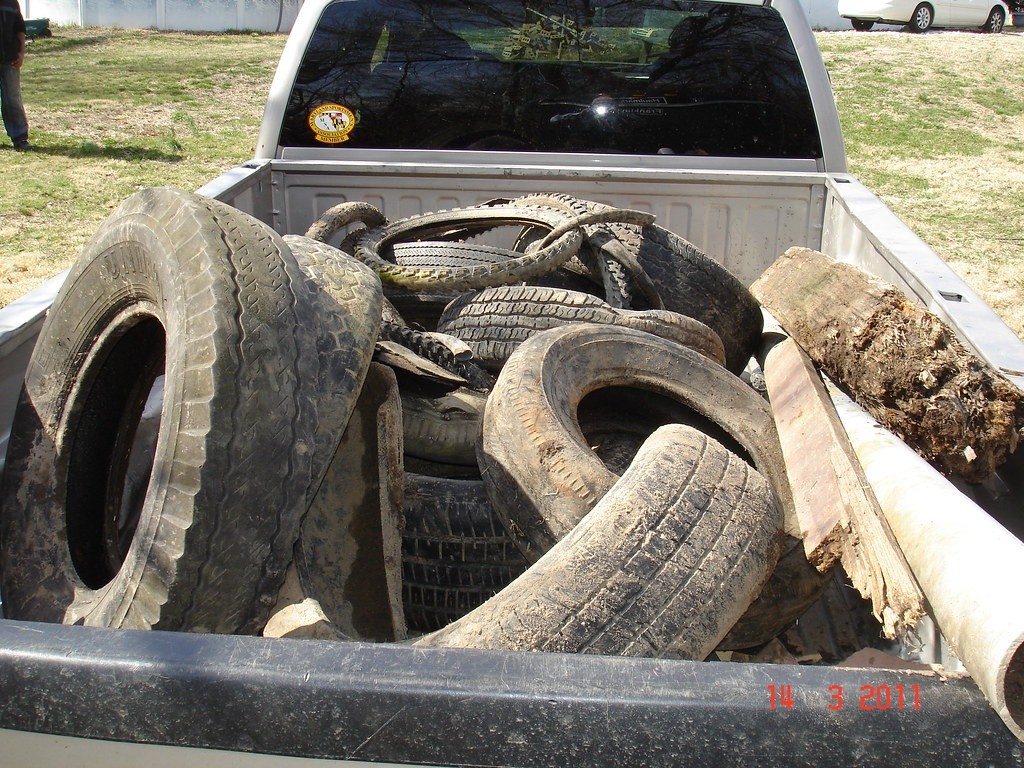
[{"xmin": 0, "ymin": 0, "xmax": 30, "ymax": 150}]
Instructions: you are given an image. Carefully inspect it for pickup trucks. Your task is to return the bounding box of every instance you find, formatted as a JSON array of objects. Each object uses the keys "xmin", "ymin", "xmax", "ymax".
[{"xmin": 0, "ymin": 0, "xmax": 1022, "ymax": 768}]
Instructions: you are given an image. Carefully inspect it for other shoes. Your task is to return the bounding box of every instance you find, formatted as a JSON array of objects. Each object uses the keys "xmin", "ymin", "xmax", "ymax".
[{"xmin": 14, "ymin": 139, "xmax": 31, "ymax": 151}]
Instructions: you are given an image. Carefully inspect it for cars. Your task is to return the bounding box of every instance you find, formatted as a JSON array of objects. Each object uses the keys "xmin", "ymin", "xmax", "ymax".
[{"xmin": 836, "ymin": 0, "xmax": 1015, "ymax": 34}]
